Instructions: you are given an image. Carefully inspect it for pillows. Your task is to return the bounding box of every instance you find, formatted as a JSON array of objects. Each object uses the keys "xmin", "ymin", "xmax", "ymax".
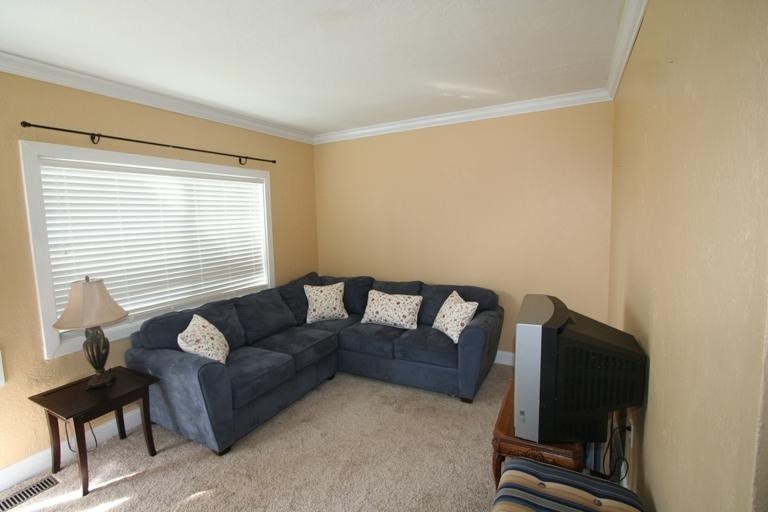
[
  {"xmin": 304, "ymin": 281, "xmax": 349, "ymax": 324},
  {"xmin": 361, "ymin": 289, "xmax": 423, "ymax": 330},
  {"xmin": 176, "ymin": 314, "xmax": 230, "ymax": 365},
  {"xmin": 432, "ymin": 290, "xmax": 479, "ymax": 344}
]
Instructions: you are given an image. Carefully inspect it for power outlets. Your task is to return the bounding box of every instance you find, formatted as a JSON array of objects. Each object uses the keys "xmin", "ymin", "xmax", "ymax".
[{"xmin": 625, "ymin": 417, "xmax": 633, "ymax": 448}]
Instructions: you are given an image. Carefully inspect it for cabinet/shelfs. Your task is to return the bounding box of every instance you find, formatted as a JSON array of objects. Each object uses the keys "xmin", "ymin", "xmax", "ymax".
[{"xmin": 491, "ymin": 374, "xmax": 587, "ymax": 490}]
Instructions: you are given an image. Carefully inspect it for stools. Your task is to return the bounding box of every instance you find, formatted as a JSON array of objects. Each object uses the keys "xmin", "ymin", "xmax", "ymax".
[{"xmin": 489, "ymin": 455, "xmax": 645, "ymax": 512}]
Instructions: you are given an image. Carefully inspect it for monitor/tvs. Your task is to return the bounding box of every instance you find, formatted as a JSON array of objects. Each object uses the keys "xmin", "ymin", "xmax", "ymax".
[{"xmin": 514, "ymin": 293, "xmax": 647, "ymax": 445}]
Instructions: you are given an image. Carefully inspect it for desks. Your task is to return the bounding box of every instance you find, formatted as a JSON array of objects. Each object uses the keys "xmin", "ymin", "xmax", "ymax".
[{"xmin": 27, "ymin": 365, "xmax": 161, "ymax": 497}]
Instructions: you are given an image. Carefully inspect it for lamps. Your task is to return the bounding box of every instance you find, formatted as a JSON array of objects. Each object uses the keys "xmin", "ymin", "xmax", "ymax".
[{"xmin": 51, "ymin": 275, "xmax": 130, "ymax": 389}]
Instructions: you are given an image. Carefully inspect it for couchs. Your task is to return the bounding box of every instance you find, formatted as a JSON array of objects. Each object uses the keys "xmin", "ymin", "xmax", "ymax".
[{"xmin": 124, "ymin": 270, "xmax": 505, "ymax": 456}]
